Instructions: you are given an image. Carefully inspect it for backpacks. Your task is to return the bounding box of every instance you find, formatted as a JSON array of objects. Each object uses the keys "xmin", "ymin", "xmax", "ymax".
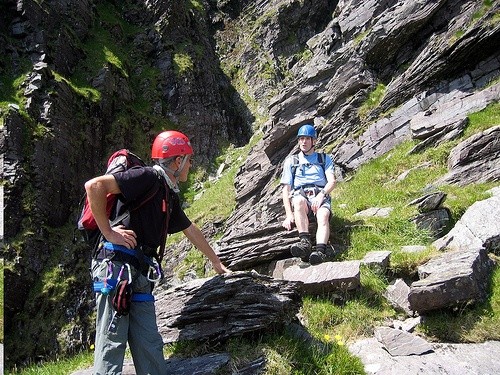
[{"xmin": 78, "ymin": 149, "xmax": 149, "ymax": 248}]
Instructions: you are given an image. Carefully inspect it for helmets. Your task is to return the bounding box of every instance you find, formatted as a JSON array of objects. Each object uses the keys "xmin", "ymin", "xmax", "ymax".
[
  {"xmin": 151, "ymin": 130, "xmax": 193, "ymax": 159},
  {"xmin": 298, "ymin": 124, "xmax": 317, "ymax": 139}
]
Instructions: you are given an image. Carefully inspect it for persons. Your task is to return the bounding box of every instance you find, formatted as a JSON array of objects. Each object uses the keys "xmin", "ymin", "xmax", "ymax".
[
  {"xmin": 84, "ymin": 131, "xmax": 232, "ymax": 375},
  {"xmin": 280, "ymin": 124, "xmax": 336, "ymax": 265}
]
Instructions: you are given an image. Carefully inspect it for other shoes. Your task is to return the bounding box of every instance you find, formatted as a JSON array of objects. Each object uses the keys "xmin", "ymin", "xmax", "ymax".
[
  {"xmin": 309, "ymin": 251, "xmax": 326, "ymax": 264},
  {"xmin": 291, "ymin": 241, "xmax": 311, "ymax": 263}
]
